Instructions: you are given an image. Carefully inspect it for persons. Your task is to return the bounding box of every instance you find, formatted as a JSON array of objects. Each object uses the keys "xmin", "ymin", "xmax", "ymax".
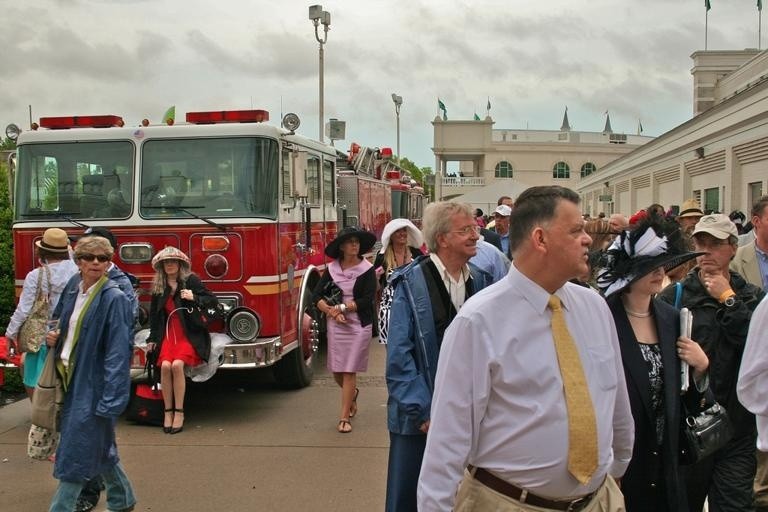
[
  {"xmin": 312, "ymin": 228, "xmax": 377, "ymax": 433},
  {"xmin": 375, "ymin": 195, "xmax": 766, "ymax": 352},
  {"xmin": 736, "ymin": 293, "xmax": 768, "ymax": 512},
  {"xmin": 77, "ymin": 228, "xmax": 140, "ymax": 512},
  {"xmin": 416, "ymin": 185, "xmax": 636, "ymax": 512},
  {"xmin": 656, "ymin": 214, "xmax": 767, "ymax": 512},
  {"xmin": 5, "ymin": 228, "xmax": 79, "ymax": 461},
  {"xmin": 386, "ymin": 200, "xmax": 497, "ymax": 512},
  {"xmin": 46, "ymin": 236, "xmax": 136, "ymax": 512},
  {"xmin": 146, "ymin": 246, "xmax": 219, "ymax": 434},
  {"xmin": 596, "ymin": 218, "xmax": 712, "ymax": 512}
]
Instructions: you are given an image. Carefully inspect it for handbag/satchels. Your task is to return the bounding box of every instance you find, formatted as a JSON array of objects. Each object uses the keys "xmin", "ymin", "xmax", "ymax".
[
  {"xmin": 27, "ymin": 423, "xmax": 61, "ymax": 460},
  {"xmin": 16, "ymin": 297, "xmax": 50, "ymax": 355},
  {"xmin": 680, "ymin": 398, "xmax": 736, "ymax": 465},
  {"xmin": 183, "ymin": 300, "xmax": 222, "ymax": 329},
  {"xmin": 321, "ymin": 281, "xmax": 344, "ymax": 306},
  {"xmin": 124, "ymin": 380, "xmax": 168, "ymax": 428},
  {"xmin": 30, "ymin": 345, "xmax": 66, "ymax": 432}
]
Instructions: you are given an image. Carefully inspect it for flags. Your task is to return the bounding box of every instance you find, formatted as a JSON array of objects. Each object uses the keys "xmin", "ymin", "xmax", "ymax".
[
  {"xmin": 640, "ymin": 124, "xmax": 643, "ymax": 132},
  {"xmin": 757, "ymin": 0, "xmax": 762, "ymax": 11},
  {"xmin": 705, "ymin": 0, "xmax": 711, "ymax": 11},
  {"xmin": 444, "ymin": 109, "xmax": 447, "ymax": 116},
  {"xmin": 439, "ymin": 101, "xmax": 445, "ymax": 110},
  {"xmin": 474, "ymin": 114, "xmax": 480, "ymax": 120},
  {"xmin": 487, "ymin": 99, "xmax": 491, "ymax": 110}
]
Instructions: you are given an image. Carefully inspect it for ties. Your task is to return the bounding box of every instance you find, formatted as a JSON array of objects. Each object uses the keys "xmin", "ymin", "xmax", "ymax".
[{"xmin": 547, "ymin": 293, "xmax": 599, "ymax": 485}]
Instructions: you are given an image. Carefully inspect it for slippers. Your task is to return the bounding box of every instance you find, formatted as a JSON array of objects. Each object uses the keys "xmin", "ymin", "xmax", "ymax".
[
  {"xmin": 347, "ymin": 387, "xmax": 359, "ymax": 417},
  {"xmin": 338, "ymin": 420, "xmax": 354, "ymax": 433}
]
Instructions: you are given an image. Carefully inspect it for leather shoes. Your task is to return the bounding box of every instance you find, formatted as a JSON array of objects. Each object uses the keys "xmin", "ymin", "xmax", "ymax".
[{"xmin": 74, "ymin": 481, "xmax": 105, "ymax": 512}]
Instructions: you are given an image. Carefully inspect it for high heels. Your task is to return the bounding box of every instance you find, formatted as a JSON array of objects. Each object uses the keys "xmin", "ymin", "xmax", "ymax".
[
  {"xmin": 170, "ymin": 407, "xmax": 185, "ymax": 435},
  {"xmin": 163, "ymin": 407, "xmax": 175, "ymax": 434}
]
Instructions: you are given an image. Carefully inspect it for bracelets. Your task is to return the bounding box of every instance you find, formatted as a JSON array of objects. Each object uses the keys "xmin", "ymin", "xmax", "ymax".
[{"xmin": 335, "ymin": 302, "xmax": 354, "ymax": 312}]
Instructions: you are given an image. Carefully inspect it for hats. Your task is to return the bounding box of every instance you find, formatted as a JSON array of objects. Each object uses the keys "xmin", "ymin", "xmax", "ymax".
[
  {"xmin": 151, "ymin": 245, "xmax": 192, "ymax": 273},
  {"xmin": 491, "ymin": 204, "xmax": 512, "ymax": 216},
  {"xmin": 34, "ymin": 227, "xmax": 72, "ymax": 252},
  {"xmin": 690, "ymin": 213, "xmax": 740, "ymax": 240},
  {"xmin": 602, "ymin": 227, "xmax": 708, "ymax": 297},
  {"xmin": 378, "ymin": 217, "xmax": 424, "ymax": 255},
  {"xmin": 323, "ymin": 225, "xmax": 377, "ymax": 260},
  {"xmin": 83, "ymin": 226, "xmax": 117, "ymax": 250},
  {"xmin": 675, "ymin": 198, "xmax": 705, "ymax": 221}
]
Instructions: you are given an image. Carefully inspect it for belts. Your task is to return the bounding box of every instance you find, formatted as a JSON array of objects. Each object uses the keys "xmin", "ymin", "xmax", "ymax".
[{"xmin": 466, "ymin": 463, "xmax": 607, "ymax": 512}]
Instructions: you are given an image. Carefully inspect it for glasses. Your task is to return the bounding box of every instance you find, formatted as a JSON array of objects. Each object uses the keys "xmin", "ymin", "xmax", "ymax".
[
  {"xmin": 441, "ymin": 224, "xmax": 481, "ymax": 235},
  {"xmin": 78, "ymin": 253, "xmax": 111, "ymax": 263}
]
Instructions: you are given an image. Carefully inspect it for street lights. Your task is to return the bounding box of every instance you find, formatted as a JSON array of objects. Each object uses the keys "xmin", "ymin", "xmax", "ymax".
[
  {"xmin": 307, "ymin": 5, "xmax": 331, "ymax": 148},
  {"xmin": 389, "ymin": 90, "xmax": 404, "ymax": 153}
]
